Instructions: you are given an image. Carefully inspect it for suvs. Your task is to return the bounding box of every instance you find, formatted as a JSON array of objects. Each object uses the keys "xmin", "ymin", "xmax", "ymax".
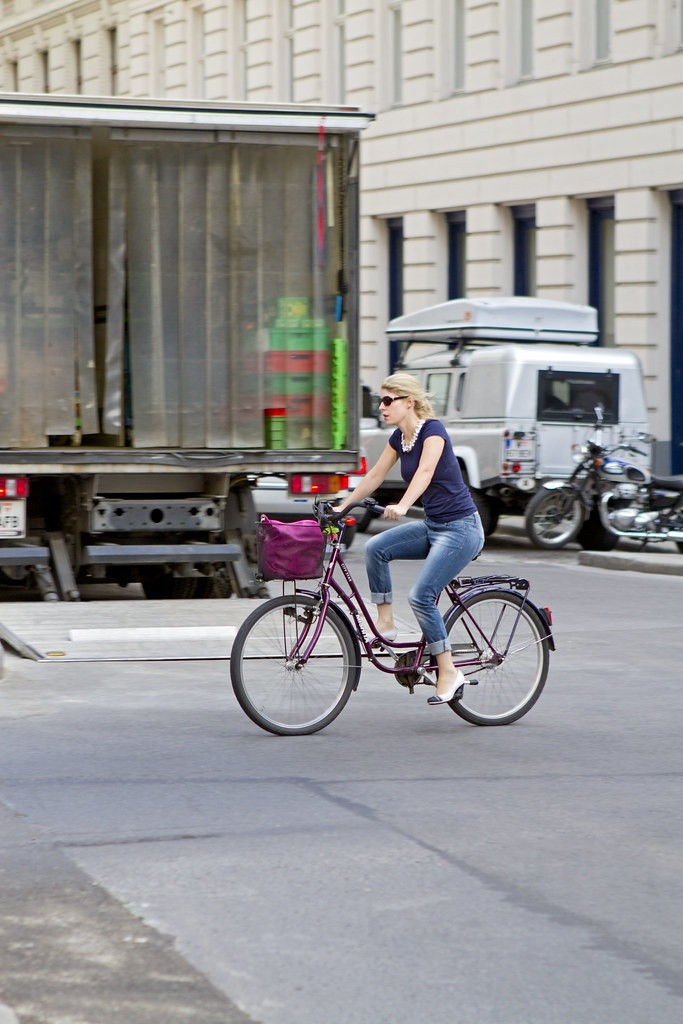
[{"xmin": 368, "ymin": 297, "xmax": 652, "ymax": 552}]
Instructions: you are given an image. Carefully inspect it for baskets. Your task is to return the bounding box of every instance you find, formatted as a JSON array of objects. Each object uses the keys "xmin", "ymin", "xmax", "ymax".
[{"xmin": 254, "ymin": 521, "xmax": 324, "ymax": 580}]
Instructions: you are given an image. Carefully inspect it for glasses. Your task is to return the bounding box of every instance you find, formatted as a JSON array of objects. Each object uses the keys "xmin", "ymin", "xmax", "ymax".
[{"xmin": 378, "ymin": 395, "xmax": 410, "ymax": 407}]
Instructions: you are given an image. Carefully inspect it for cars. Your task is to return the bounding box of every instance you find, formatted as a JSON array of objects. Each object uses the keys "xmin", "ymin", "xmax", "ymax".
[{"xmin": 249, "ymin": 426, "xmax": 368, "ymax": 552}]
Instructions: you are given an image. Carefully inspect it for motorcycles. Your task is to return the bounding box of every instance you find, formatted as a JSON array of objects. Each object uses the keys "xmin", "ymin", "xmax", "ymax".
[{"xmin": 523, "ymin": 398, "xmax": 683, "ymax": 555}]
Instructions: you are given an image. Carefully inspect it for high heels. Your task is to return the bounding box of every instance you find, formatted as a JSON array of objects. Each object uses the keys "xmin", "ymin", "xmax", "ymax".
[
  {"xmin": 364, "ymin": 630, "xmax": 397, "ymax": 652},
  {"xmin": 427, "ymin": 668, "xmax": 465, "ymax": 705}
]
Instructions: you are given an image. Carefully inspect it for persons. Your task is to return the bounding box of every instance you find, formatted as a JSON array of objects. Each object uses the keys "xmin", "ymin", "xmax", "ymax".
[{"xmin": 331, "ymin": 373, "xmax": 485, "ymax": 705}]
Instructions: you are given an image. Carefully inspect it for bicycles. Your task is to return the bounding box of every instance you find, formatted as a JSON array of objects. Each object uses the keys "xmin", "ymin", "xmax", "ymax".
[{"xmin": 229, "ymin": 492, "xmax": 557, "ymax": 736}]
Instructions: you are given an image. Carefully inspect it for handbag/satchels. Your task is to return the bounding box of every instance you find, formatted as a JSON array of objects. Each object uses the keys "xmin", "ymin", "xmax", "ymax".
[{"xmin": 258, "ymin": 514, "xmax": 324, "ymax": 579}]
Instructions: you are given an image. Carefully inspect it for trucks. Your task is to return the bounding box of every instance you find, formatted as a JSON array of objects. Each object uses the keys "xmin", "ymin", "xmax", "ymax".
[{"xmin": 0, "ymin": 90, "xmax": 377, "ymax": 604}]
